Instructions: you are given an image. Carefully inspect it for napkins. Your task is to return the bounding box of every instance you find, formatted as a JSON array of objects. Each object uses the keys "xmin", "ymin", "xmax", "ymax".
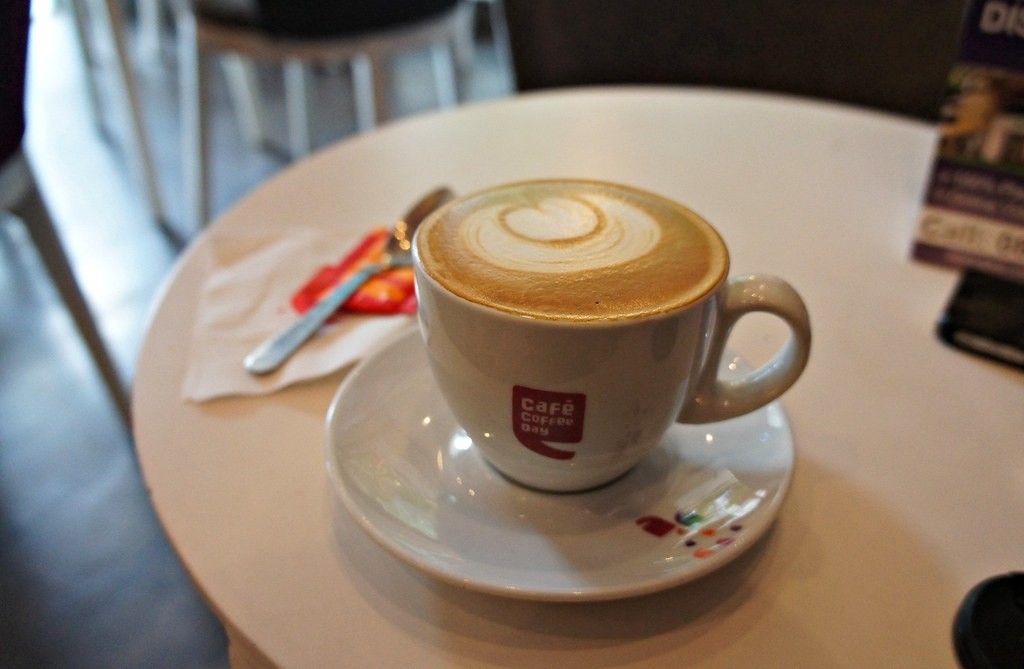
[{"xmin": 174, "ymin": 227, "xmax": 415, "ymax": 404}]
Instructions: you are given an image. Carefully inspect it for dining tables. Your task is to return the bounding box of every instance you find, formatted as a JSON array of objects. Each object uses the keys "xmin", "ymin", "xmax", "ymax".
[{"xmin": 130, "ymin": 84, "xmax": 1024, "ymax": 669}]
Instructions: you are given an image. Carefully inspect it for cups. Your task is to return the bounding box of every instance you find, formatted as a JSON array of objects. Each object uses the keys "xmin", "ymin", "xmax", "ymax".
[{"xmin": 412, "ymin": 178, "xmax": 811, "ymax": 492}]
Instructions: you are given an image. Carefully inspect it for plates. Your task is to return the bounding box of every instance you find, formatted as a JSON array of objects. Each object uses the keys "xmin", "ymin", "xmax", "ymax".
[
  {"xmin": 325, "ymin": 325, "xmax": 795, "ymax": 602},
  {"xmin": 192, "ymin": 0, "xmax": 462, "ymax": 54},
  {"xmin": 951, "ymin": 558, "xmax": 1024, "ymax": 669}
]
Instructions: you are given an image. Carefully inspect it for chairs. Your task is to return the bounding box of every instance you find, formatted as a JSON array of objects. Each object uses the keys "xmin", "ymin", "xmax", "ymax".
[
  {"xmin": 487, "ymin": 0, "xmax": 964, "ymax": 125},
  {"xmin": 0, "ymin": 0, "xmax": 481, "ymax": 439}
]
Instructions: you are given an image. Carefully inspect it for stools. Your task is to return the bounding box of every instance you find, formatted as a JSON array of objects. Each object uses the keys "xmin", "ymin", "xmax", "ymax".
[{"xmin": 171, "ymin": 0, "xmax": 473, "ymax": 237}]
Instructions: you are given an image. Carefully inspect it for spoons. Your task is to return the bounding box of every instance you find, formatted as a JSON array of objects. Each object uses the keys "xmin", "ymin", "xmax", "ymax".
[{"xmin": 244, "ymin": 188, "xmax": 458, "ymax": 375}]
